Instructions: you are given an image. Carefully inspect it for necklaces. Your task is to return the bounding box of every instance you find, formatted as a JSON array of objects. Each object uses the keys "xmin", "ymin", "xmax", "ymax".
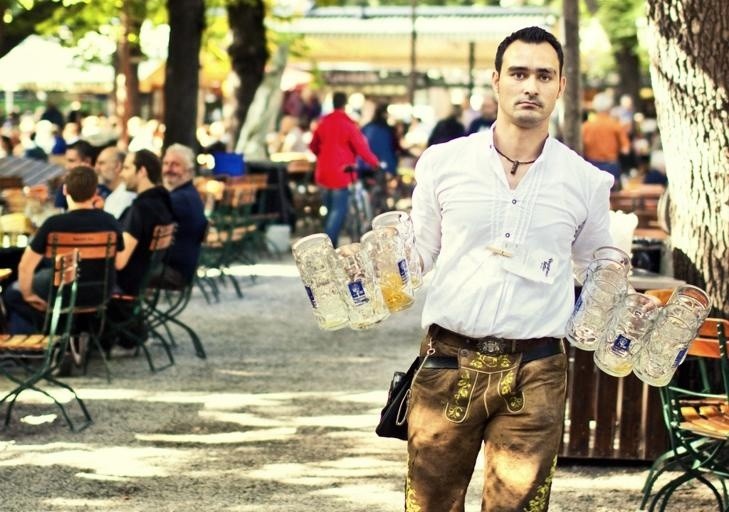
[{"xmin": 496, "ymin": 149, "xmax": 538, "ymax": 174}]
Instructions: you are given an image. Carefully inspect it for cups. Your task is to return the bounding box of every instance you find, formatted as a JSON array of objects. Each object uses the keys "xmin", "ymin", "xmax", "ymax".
[
  {"xmin": 564, "ymin": 246, "xmax": 631, "ymax": 351},
  {"xmin": 593, "ymin": 293, "xmax": 659, "ymax": 377},
  {"xmin": 327, "ymin": 243, "xmax": 392, "ymax": 330},
  {"xmin": 291, "ymin": 233, "xmax": 349, "ymax": 332},
  {"xmin": 360, "ymin": 227, "xmax": 416, "ymax": 312},
  {"xmin": 371, "ymin": 211, "xmax": 423, "ymax": 292},
  {"xmin": 632, "ymin": 284, "xmax": 712, "ymax": 386}
]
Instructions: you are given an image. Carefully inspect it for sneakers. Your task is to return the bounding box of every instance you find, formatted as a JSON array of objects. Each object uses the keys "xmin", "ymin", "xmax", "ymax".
[
  {"xmin": 50, "ymin": 341, "xmax": 61, "ymax": 375},
  {"xmin": 111, "ymin": 345, "xmax": 136, "ymax": 357},
  {"xmin": 70, "ymin": 335, "xmax": 82, "ymax": 366}
]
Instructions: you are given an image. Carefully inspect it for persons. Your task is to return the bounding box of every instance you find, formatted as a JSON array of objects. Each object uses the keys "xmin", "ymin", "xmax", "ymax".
[
  {"xmin": 310, "ymin": 92, "xmax": 382, "ymax": 248},
  {"xmin": 358, "ymin": 102, "xmax": 397, "ymax": 175},
  {"xmin": 424, "ymin": 105, "xmax": 467, "ymax": 147},
  {"xmin": 95, "ymin": 148, "xmax": 139, "ymax": 218},
  {"xmin": 339, "ymin": 25, "xmax": 662, "ymax": 512},
  {"xmin": 57, "ymin": 141, "xmax": 111, "ymax": 207},
  {"xmin": 581, "ymin": 93, "xmax": 630, "ymax": 192},
  {"xmin": 161, "ymin": 146, "xmax": 206, "ymax": 289},
  {"xmin": 112, "ymin": 150, "xmax": 175, "ymax": 295},
  {"xmin": 467, "ymin": 96, "xmax": 499, "ymax": 134},
  {"xmin": 4, "ymin": 166, "xmax": 124, "ymax": 329}
]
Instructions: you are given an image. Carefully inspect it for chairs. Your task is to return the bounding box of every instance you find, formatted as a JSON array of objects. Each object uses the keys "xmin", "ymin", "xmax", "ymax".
[
  {"xmin": 285, "ymin": 157, "xmax": 319, "ymax": 235},
  {"xmin": 41, "ymin": 231, "xmax": 116, "ymax": 386},
  {"xmin": 213, "ymin": 172, "xmax": 283, "ymax": 269},
  {"xmin": 106, "ymin": 222, "xmax": 179, "ymax": 373},
  {"xmin": 150, "ymin": 186, "xmax": 237, "ymax": 362},
  {"xmin": 641, "ymin": 285, "xmax": 727, "ymax": 512},
  {"xmin": 647, "ymin": 319, "xmax": 728, "ymax": 512},
  {"xmin": 1, "ymin": 176, "xmax": 25, "ymax": 200},
  {"xmin": 0, "ymin": 248, "xmax": 92, "ymax": 435}
]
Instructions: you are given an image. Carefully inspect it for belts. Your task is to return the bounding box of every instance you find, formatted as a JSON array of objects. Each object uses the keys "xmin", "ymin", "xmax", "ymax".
[{"xmin": 427, "ymin": 324, "xmax": 564, "ymax": 361}]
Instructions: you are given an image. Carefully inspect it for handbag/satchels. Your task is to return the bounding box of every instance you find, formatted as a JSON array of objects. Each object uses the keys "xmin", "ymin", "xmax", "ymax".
[{"xmin": 373, "ymin": 357, "xmax": 419, "ymax": 440}]
